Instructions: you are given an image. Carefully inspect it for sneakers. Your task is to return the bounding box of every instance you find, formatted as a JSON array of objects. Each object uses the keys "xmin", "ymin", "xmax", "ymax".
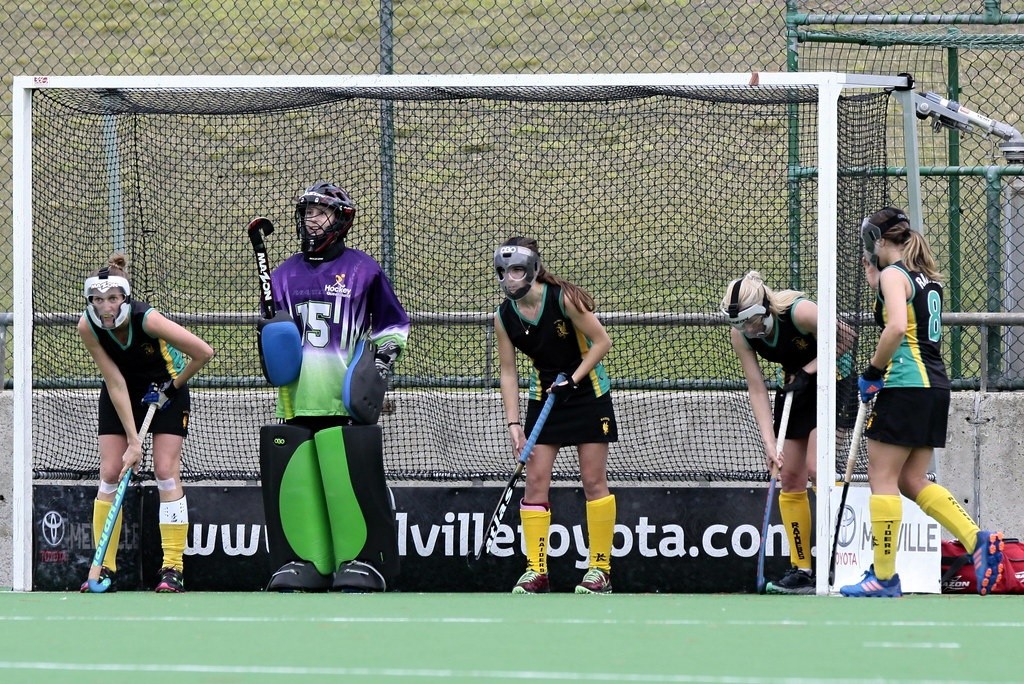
[
  {"xmin": 155, "ymin": 564, "xmax": 186, "ymax": 593},
  {"xmin": 839, "ymin": 563, "xmax": 903, "ymax": 598},
  {"xmin": 964, "ymin": 530, "xmax": 1004, "ymax": 596},
  {"xmin": 575, "ymin": 569, "xmax": 613, "ymax": 594},
  {"xmin": 512, "ymin": 570, "xmax": 551, "ymax": 594},
  {"xmin": 765, "ymin": 565, "xmax": 816, "ymax": 595},
  {"xmin": 80, "ymin": 565, "xmax": 119, "ymax": 593}
]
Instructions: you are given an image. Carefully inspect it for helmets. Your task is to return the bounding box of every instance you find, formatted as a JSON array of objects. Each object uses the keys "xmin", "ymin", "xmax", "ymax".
[{"xmin": 294, "ymin": 182, "xmax": 356, "ymax": 260}]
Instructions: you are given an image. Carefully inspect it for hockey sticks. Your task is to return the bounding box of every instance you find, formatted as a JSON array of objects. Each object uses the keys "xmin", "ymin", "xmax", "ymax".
[
  {"xmin": 88, "ymin": 383, "xmax": 161, "ymax": 593},
  {"xmin": 758, "ymin": 392, "xmax": 795, "ymax": 595},
  {"xmin": 466, "ymin": 372, "xmax": 569, "ymax": 572},
  {"xmin": 828, "ymin": 399, "xmax": 869, "ymax": 587},
  {"xmin": 247, "ymin": 219, "xmax": 296, "ymax": 421}
]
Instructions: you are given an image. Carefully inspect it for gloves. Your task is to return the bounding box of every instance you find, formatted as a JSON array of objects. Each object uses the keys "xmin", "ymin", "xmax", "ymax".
[
  {"xmin": 139, "ymin": 378, "xmax": 178, "ymax": 412},
  {"xmin": 774, "ymin": 367, "xmax": 812, "ymax": 400},
  {"xmin": 857, "ymin": 364, "xmax": 885, "ymax": 403}
]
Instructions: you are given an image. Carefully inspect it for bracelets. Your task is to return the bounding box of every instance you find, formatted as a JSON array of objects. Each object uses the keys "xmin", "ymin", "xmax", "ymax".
[{"xmin": 508, "ymin": 422, "xmax": 520, "ymax": 427}]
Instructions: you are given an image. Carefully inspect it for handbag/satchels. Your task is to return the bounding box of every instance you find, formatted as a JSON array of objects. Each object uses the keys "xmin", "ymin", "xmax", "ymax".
[{"xmin": 941, "ymin": 538, "xmax": 1024, "ymax": 594}]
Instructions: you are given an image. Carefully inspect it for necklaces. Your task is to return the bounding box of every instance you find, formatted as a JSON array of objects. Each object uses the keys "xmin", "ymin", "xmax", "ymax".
[{"xmin": 516, "ymin": 290, "xmax": 542, "ymax": 335}]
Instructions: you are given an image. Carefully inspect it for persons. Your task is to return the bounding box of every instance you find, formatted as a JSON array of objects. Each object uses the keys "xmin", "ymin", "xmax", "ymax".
[
  {"xmin": 494, "ymin": 236, "xmax": 619, "ymax": 594},
  {"xmin": 77, "ymin": 253, "xmax": 214, "ymax": 593},
  {"xmin": 719, "ymin": 270, "xmax": 857, "ymax": 596},
  {"xmin": 840, "ymin": 207, "xmax": 1005, "ymax": 597},
  {"xmin": 256, "ymin": 183, "xmax": 411, "ymax": 593}
]
